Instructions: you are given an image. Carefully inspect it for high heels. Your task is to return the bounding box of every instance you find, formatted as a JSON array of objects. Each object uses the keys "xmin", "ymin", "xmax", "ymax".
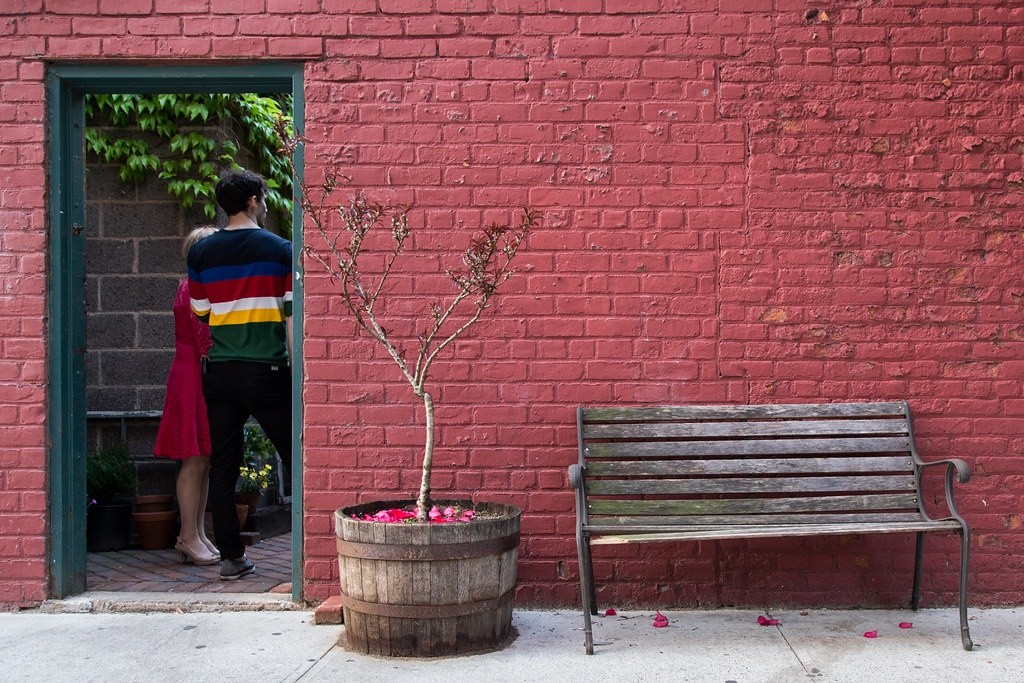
[{"xmin": 174, "ymin": 537, "xmax": 223, "ymax": 564}]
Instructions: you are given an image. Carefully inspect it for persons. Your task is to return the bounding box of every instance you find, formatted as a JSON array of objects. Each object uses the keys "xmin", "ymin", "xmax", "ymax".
[
  {"xmin": 187, "ymin": 170, "xmax": 292, "ymax": 580},
  {"xmin": 153, "ymin": 226, "xmax": 220, "ymax": 565}
]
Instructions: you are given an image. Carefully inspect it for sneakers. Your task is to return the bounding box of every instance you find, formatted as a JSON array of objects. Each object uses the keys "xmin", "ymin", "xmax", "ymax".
[{"xmin": 219, "ymin": 556, "xmax": 255, "ymax": 579}]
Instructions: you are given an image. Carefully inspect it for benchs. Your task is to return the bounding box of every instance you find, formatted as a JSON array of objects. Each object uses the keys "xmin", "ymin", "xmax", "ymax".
[{"xmin": 563, "ymin": 399, "xmax": 973, "ymax": 656}]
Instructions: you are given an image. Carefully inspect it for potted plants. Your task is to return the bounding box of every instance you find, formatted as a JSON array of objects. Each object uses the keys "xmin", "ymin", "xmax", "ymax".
[{"xmin": 274, "ymin": 113, "xmax": 548, "ymax": 658}]
